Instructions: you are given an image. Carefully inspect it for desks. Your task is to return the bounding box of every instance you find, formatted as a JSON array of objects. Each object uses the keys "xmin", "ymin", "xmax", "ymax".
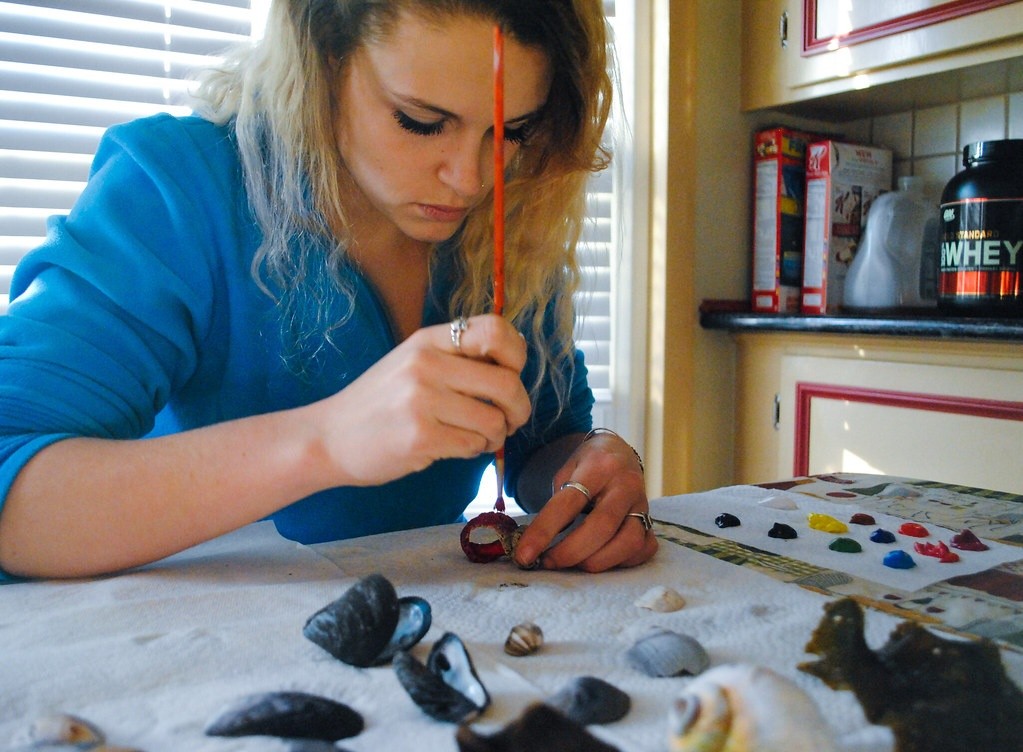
[{"xmin": 1, "ymin": 472, "xmax": 1023, "ymax": 752}]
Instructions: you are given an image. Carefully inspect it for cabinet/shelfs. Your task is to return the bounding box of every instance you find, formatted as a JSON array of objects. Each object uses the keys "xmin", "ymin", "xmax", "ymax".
[
  {"xmin": 742, "ymin": 0, "xmax": 1023, "ymax": 120},
  {"xmin": 727, "ymin": 330, "xmax": 1022, "ymax": 498}
]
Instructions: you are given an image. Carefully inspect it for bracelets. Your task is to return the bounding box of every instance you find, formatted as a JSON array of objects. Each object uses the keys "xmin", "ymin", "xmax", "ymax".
[{"xmin": 583, "ymin": 427, "xmax": 645, "ymax": 474}]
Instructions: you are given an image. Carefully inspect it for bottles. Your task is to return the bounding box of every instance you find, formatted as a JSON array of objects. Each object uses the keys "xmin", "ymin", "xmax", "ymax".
[{"xmin": 839, "ymin": 175, "xmax": 939, "ymax": 317}]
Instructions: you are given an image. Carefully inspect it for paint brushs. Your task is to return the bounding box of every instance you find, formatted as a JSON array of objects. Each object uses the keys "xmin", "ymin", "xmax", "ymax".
[{"xmin": 489, "ymin": 21, "xmax": 506, "ymax": 510}]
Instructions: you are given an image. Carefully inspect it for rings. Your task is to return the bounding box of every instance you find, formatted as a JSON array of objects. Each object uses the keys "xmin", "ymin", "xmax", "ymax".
[
  {"xmin": 560, "ymin": 481, "xmax": 592, "ymax": 501},
  {"xmin": 451, "ymin": 316, "xmax": 468, "ymax": 353},
  {"xmin": 626, "ymin": 512, "xmax": 654, "ymax": 531}
]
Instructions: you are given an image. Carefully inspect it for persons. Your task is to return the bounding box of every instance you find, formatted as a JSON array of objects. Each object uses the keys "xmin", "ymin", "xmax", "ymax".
[{"xmin": 0, "ymin": 0, "xmax": 659, "ymax": 579}]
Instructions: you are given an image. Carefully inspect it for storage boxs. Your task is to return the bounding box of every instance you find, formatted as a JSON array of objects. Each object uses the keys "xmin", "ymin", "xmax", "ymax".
[{"xmin": 750, "ymin": 126, "xmax": 894, "ymax": 316}]
